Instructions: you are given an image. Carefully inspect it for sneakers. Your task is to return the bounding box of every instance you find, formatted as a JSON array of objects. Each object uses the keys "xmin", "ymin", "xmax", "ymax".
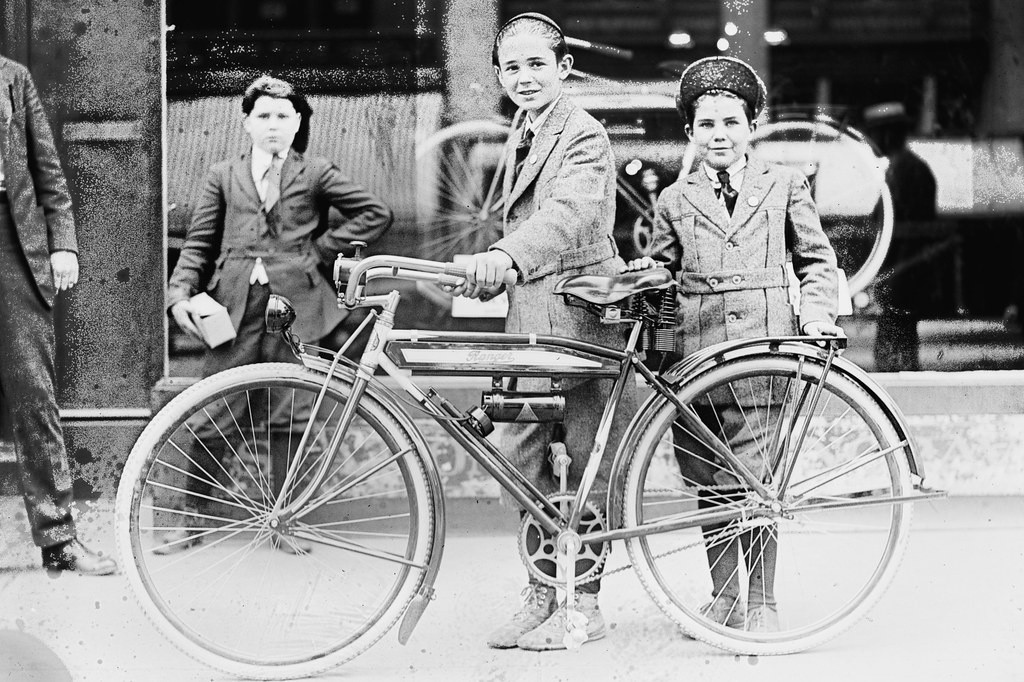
[
  {"xmin": 487, "ymin": 584, "xmax": 558, "ymax": 647},
  {"xmin": 517, "ymin": 593, "xmax": 605, "ymax": 650}
]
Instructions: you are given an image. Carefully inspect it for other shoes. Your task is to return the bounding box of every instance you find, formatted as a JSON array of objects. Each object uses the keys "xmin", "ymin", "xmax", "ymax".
[
  {"xmin": 679, "ymin": 596, "xmax": 739, "ymax": 640},
  {"xmin": 747, "ymin": 607, "xmax": 778, "ymax": 633},
  {"xmin": 271, "ymin": 511, "xmax": 312, "ymax": 554},
  {"xmin": 153, "ymin": 506, "xmax": 205, "ymax": 555}
]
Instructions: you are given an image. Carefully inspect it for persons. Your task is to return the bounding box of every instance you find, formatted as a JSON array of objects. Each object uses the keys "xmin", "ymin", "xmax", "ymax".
[
  {"xmin": 620, "ymin": 56, "xmax": 850, "ymax": 640},
  {"xmin": 434, "ymin": 12, "xmax": 637, "ymax": 651},
  {"xmin": 0, "ymin": 56, "xmax": 119, "ymax": 577},
  {"xmin": 864, "ymin": 104, "xmax": 939, "ymax": 374},
  {"xmin": 153, "ymin": 75, "xmax": 396, "ymax": 557}
]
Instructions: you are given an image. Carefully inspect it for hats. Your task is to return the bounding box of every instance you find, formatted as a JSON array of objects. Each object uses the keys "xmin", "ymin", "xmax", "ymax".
[
  {"xmin": 675, "ymin": 56, "xmax": 767, "ymax": 125},
  {"xmin": 492, "ymin": 12, "xmax": 564, "ymax": 67}
]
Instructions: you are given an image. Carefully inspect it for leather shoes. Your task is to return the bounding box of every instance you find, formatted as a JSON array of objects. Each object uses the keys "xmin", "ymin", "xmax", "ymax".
[{"xmin": 42, "ymin": 538, "xmax": 117, "ymax": 575}]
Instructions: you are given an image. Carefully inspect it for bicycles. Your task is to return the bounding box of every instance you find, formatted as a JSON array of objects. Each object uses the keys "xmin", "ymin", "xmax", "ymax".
[
  {"xmin": 114, "ymin": 237, "xmax": 948, "ymax": 682},
  {"xmin": 407, "ymin": 33, "xmax": 898, "ymax": 348}
]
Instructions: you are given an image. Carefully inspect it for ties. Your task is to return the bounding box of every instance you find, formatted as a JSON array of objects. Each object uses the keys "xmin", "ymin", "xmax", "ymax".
[
  {"xmin": 511, "ymin": 128, "xmax": 535, "ymax": 193},
  {"xmin": 265, "ymin": 154, "xmax": 281, "ymax": 213}
]
